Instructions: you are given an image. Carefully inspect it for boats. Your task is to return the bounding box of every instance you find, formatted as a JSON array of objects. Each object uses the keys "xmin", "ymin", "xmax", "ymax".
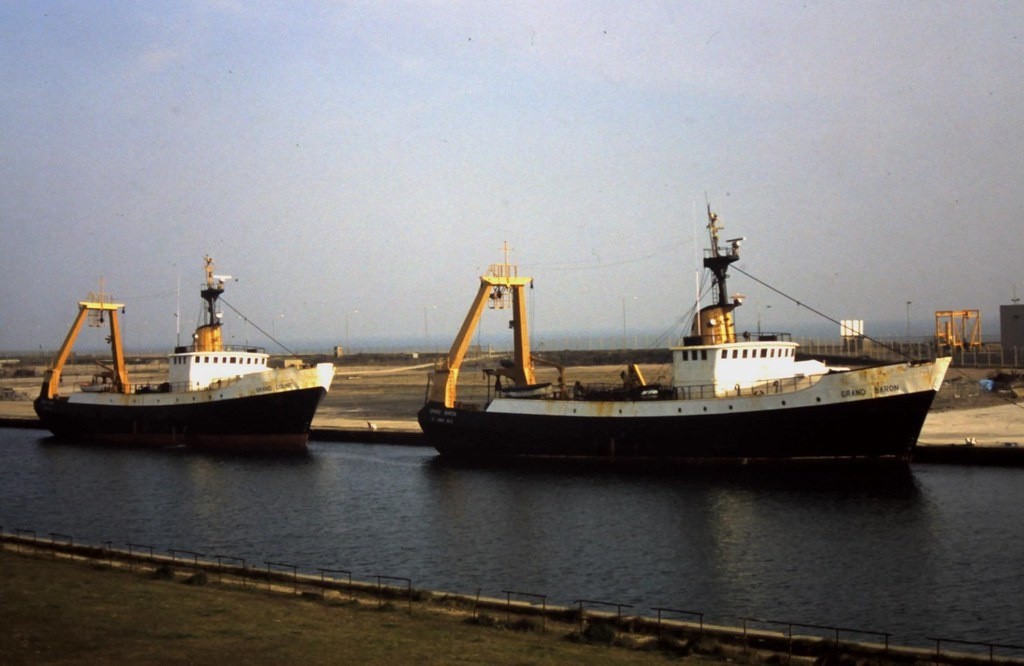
[
  {"xmin": 413, "ymin": 203, "xmax": 954, "ymax": 459},
  {"xmin": 32, "ymin": 249, "xmax": 336, "ymax": 450}
]
